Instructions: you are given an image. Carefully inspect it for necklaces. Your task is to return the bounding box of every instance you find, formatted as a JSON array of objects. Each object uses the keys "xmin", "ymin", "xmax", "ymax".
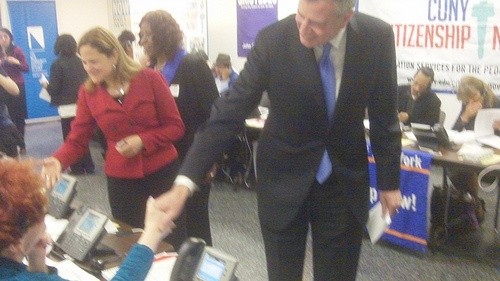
[{"xmin": 114, "ymin": 89, "xmax": 125, "ymax": 106}]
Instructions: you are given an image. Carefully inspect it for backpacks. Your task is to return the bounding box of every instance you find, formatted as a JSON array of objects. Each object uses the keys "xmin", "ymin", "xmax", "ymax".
[{"xmin": 431, "ymin": 186, "xmax": 481, "ymax": 252}]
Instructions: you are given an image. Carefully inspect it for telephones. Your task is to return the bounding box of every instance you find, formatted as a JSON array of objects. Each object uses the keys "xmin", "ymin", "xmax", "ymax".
[
  {"xmin": 412, "ymin": 122, "xmax": 451, "ymax": 147},
  {"xmin": 57, "ymin": 205, "xmax": 109, "ymax": 261},
  {"xmin": 170, "ymin": 237, "xmax": 238, "ymax": 281},
  {"xmin": 45, "ymin": 173, "xmax": 78, "ymax": 218}
]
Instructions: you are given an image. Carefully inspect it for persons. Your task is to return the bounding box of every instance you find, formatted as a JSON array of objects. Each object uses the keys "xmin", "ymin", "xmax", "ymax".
[
  {"xmin": 36, "ymin": 29, "xmax": 188, "ymax": 252},
  {"xmin": 136, "ymin": 7, "xmax": 220, "ymax": 279},
  {"xmin": 1, "ymin": 158, "xmax": 177, "ymax": 280},
  {"xmin": 155, "ymin": 2, "xmax": 405, "ymax": 280},
  {"xmin": 2, "ymin": 27, "xmax": 30, "ymax": 155},
  {"xmin": 446, "ymin": 75, "xmax": 499, "ymax": 232},
  {"xmin": 386, "ymin": 65, "xmax": 442, "ymax": 154},
  {"xmin": 39, "ymin": 28, "xmax": 245, "ymax": 177}
]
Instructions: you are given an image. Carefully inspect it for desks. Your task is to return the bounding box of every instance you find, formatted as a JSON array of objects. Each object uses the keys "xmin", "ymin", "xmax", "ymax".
[
  {"xmin": 49, "ymin": 207, "xmax": 239, "ymax": 281},
  {"xmin": 241, "ymin": 105, "xmax": 500, "ymax": 261}
]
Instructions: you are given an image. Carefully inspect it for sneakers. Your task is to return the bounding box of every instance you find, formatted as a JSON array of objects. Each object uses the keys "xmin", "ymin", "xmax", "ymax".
[
  {"xmin": 86, "ymin": 162, "xmax": 95, "ymax": 173},
  {"xmin": 19, "ymin": 147, "xmax": 26, "ymax": 155},
  {"xmin": 67, "ymin": 166, "xmax": 84, "ymax": 175},
  {"xmin": 475, "ymin": 199, "xmax": 485, "ymax": 221}
]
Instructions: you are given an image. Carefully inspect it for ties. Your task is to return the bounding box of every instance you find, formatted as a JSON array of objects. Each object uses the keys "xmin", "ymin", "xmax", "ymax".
[{"xmin": 316, "ymin": 42, "xmax": 336, "ymax": 184}]
